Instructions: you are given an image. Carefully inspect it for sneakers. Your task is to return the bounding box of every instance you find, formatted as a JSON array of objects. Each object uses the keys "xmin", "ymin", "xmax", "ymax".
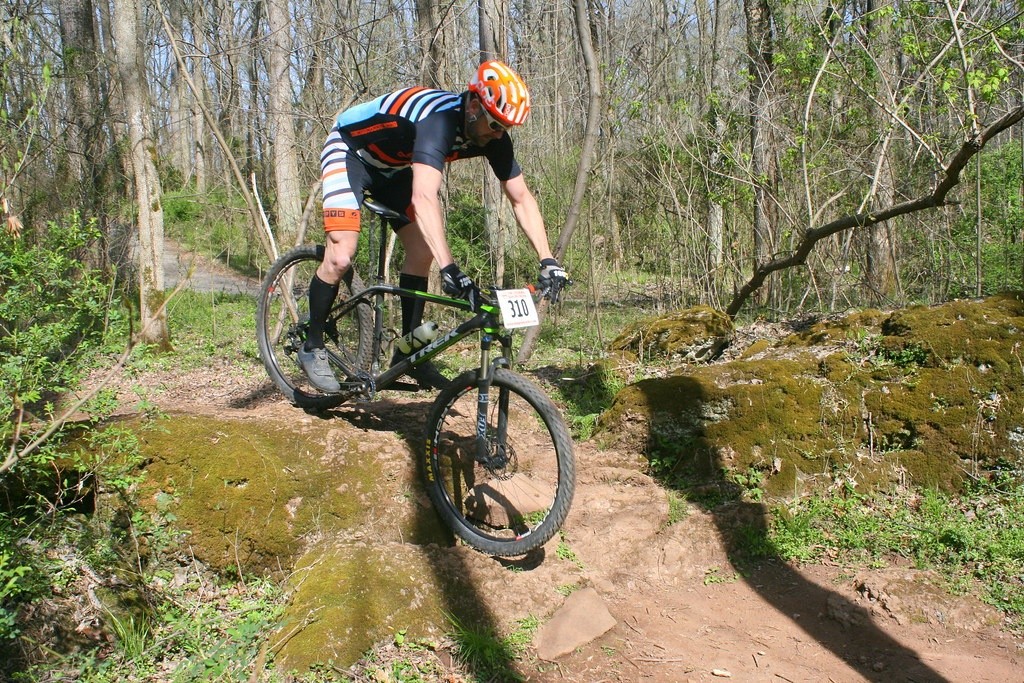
[
  {"xmin": 389, "ymin": 353, "xmax": 451, "ymax": 389},
  {"xmin": 297, "ymin": 340, "xmax": 340, "ymax": 393}
]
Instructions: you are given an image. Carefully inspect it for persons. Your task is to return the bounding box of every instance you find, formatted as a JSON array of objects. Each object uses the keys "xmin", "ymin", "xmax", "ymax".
[{"xmin": 297, "ymin": 60, "xmax": 567, "ymax": 394}]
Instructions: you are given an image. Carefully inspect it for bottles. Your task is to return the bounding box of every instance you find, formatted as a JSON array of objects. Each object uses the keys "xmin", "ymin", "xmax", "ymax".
[{"xmin": 398, "ymin": 321, "xmax": 438, "ymax": 354}]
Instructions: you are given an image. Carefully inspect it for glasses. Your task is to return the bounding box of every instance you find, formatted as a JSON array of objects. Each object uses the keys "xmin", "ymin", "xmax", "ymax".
[{"xmin": 474, "ymin": 93, "xmax": 508, "ymax": 132}]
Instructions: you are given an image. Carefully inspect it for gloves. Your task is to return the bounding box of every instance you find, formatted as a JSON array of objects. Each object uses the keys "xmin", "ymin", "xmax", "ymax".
[
  {"xmin": 440, "ymin": 263, "xmax": 478, "ymax": 314},
  {"xmin": 537, "ymin": 257, "xmax": 569, "ymax": 305}
]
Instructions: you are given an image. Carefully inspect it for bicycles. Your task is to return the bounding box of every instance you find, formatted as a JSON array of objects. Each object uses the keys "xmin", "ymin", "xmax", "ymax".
[{"xmin": 257, "ymin": 201, "xmax": 576, "ymax": 557}]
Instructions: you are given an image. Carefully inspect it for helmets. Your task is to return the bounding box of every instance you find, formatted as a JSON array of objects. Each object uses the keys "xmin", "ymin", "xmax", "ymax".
[{"xmin": 468, "ymin": 60, "xmax": 531, "ymax": 126}]
